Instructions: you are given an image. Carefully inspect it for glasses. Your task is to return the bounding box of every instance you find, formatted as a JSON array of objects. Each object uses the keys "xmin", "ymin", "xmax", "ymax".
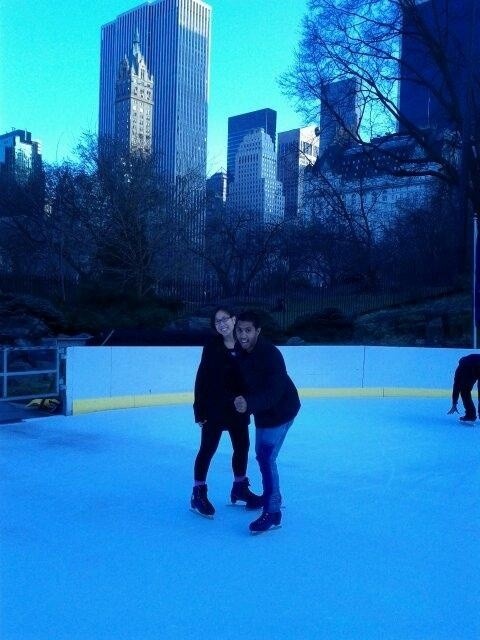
[{"xmin": 214, "ymin": 316, "xmax": 230, "ymax": 325}]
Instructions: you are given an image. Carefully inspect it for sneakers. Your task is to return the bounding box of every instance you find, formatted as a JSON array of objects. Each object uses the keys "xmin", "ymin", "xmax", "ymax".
[
  {"xmin": 231, "ymin": 478, "xmax": 260, "ymax": 504},
  {"xmin": 191, "ymin": 483, "xmax": 216, "ymax": 515},
  {"xmin": 460, "ymin": 411, "xmax": 477, "ymax": 421},
  {"xmin": 249, "ymin": 512, "xmax": 282, "ymax": 532}
]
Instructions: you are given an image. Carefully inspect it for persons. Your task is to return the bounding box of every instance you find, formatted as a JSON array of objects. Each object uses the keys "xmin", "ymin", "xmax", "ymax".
[
  {"xmin": 446, "ymin": 353, "xmax": 480, "ymax": 423},
  {"xmin": 190, "ymin": 305, "xmax": 262, "ymax": 515},
  {"xmin": 234, "ymin": 312, "xmax": 301, "ymax": 534}
]
